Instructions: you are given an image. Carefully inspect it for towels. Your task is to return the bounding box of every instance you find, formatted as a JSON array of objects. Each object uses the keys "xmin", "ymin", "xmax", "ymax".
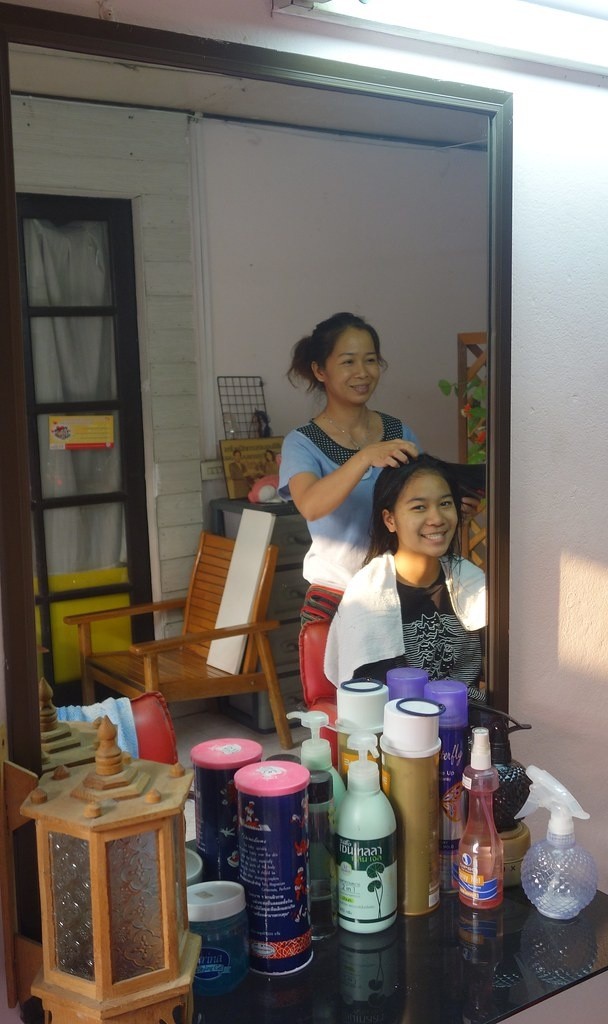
[
  {"xmin": 322, "ymin": 548, "xmax": 488, "ymax": 687},
  {"xmin": 54, "ymin": 697, "xmax": 141, "ymax": 759}
]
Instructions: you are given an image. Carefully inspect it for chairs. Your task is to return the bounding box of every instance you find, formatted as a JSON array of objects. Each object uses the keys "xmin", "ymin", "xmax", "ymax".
[
  {"xmin": 65, "ymin": 529, "xmax": 294, "ymax": 749},
  {"xmin": 295, "ymin": 619, "xmax": 338, "ymax": 765},
  {"xmin": 52, "ymin": 692, "xmax": 198, "ymax": 803}
]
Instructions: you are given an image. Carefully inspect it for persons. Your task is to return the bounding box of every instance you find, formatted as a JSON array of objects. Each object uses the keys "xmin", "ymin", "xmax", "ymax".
[
  {"xmin": 324, "ymin": 453, "xmax": 486, "ymax": 701},
  {"xmin": 229, "ymin": 449, "xmax": 279, "ymax": 497},
  {"xmin": 275, "ymin": 312, "xmax": 480, "ymax": 773}
]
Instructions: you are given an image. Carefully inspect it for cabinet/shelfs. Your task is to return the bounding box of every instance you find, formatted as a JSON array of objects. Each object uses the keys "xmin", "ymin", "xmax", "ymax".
[{"xmin": 208, "ymin": 493, "xmax": 311, "ymax": 727}]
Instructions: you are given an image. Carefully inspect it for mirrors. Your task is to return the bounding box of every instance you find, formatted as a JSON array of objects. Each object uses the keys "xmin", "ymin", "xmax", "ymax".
[{"xmin": 0, "ymin": 0, "xmax": 513, "ymax": 884}]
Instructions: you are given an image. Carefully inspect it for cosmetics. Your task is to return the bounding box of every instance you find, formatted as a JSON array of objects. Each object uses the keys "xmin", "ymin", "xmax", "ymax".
[
  {"xmin": 185, "ymin": 659, "xmax": 443, "ymax": 889},
  {"xmin": 182, "ymin": 678, "xmax": 596, "ymax": 999}
]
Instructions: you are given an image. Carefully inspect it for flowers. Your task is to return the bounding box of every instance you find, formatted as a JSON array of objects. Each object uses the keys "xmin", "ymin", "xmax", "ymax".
[{"xmin": 436, "ymin": 375, "xmax": 488, "ymax": 465}]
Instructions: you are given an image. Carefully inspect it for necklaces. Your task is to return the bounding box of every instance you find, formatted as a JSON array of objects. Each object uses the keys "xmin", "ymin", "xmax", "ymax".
[{"xmin": 322, "ymin": 410, "xmax": 369, "ymax": 451}]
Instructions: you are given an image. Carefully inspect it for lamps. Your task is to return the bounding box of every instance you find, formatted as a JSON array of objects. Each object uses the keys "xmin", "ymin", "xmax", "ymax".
[
  {"xmin": 17, "ymin": 718, "xmax": 204, "ymax": 1024},
  {"xmin": 35, "ymin": 679, "xmax": 94, "ymax": 771}
]
